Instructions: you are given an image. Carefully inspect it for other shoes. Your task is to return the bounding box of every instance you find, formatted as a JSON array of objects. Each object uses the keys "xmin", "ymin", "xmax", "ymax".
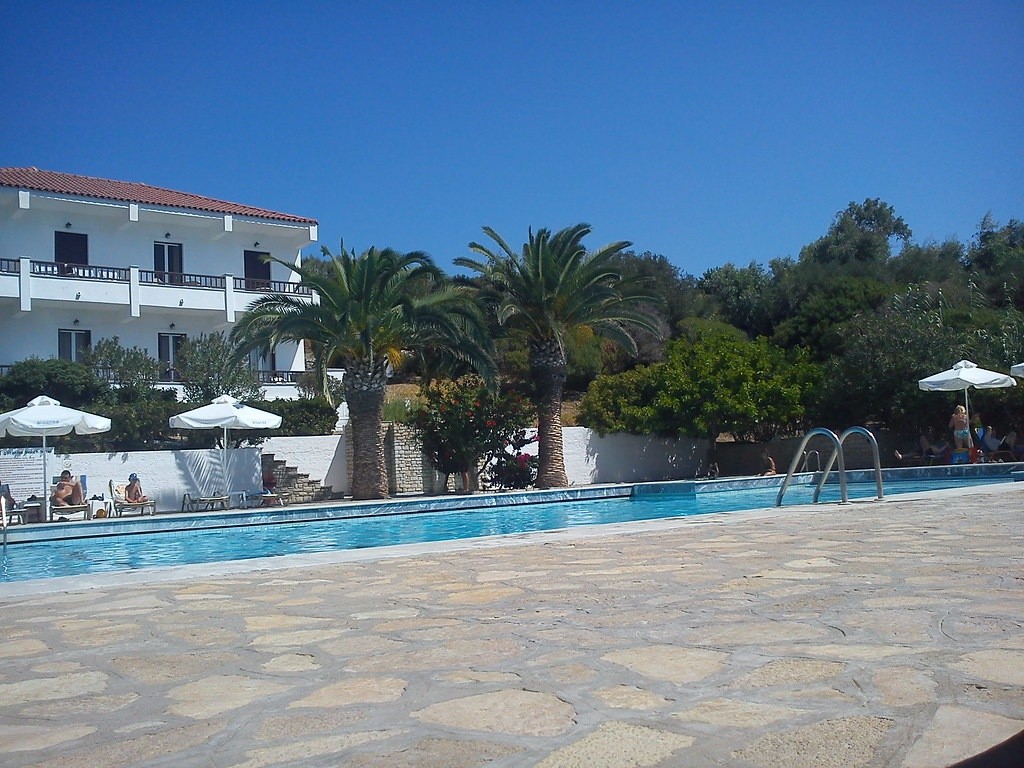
[
  {"xmin": 58, "ymin": 517, "xmax": 69, "ymax": 522},
  {"xmin": 27, "ymin": 494, "xmax": 37, "ymax": 500}
]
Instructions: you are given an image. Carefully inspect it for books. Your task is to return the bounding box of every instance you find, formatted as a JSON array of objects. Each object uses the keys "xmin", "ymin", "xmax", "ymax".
[{"xmin": 50, "ymin": 482, "xmax": 64, "ymax": 493}]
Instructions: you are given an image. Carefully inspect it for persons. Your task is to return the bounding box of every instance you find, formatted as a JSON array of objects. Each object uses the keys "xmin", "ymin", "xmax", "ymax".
[
  {"xmin": 50, "ymin": 470, "xmax": 87, "ymax": 506},
  {"xmin": 984, "ymin": 424, "xmax": 1024, "ymax": 463},
  {"xmin": 760, "ymin": 457, "xmax": 776, "ymax": 476},
  {"xmin": 125, "ymin": 473, "xmax": 148, "ymax": 503},
  {"xmin": 706, "ymin": 461, "xmax": 719, "ymax": 479},
  {"xmin": 0, "ymin": 481, "xmax": 15, "ymax": 510},
  {"xmin": 894, "ymin": 434, "xmax": 949, "ymax": 461},
  {"xmin": 949, "ymin": 406, "xmax": 973, "ymax": 449}
]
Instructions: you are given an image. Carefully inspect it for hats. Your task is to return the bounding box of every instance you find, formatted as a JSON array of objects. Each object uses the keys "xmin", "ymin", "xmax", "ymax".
[{"xmin": 129, "ymin": 473, "xmax": 138, "ymax": 480}]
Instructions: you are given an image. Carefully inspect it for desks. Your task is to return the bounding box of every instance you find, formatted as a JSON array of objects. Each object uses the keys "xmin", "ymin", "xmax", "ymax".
[
  {"xmin": 24, "ymin": 503, "xmax": 41, "ymax": 522},
  {"xmin": 92, "ymin": 498, "xmax": 114, "ymax": 519},
  {"xmin": 222, "ymin": 491, "xmax": 247, "ymax": 510}
]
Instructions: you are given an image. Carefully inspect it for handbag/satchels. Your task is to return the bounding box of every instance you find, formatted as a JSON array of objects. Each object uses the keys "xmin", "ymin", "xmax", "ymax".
[{"xmin": 93, "ymin": 509, "xmax": 106, "ymax": 519}]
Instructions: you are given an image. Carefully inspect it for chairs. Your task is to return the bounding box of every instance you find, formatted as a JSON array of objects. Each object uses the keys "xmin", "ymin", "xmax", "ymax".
[
  {"xmin": 975, "ymin": 428, "xmax": 1017, "ymax": 462},
  {"xmin": 181, "ymin": 492, "xmax": 229, "ymax": 512},
  {"xmin": 109, "ymin": 480, "xmax": 156, "ymax": 515},
  {"xmin": 50, "ymin": 475, "xmax": 90, "ymax": 522},
  {"xmin": 0, "ymin": 484, "xmax": 27, "ymax": 525},
  {"xmin": 903, "ymin": 437, "xmax": 950, "ymax": 467},
  {"xmin": 243, "ymin": 488, "xmax": 290, "ymax": 507}
]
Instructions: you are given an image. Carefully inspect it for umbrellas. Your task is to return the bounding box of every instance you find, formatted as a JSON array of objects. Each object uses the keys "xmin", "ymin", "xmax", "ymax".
[
  {"xmin": 1011, "ymin": 362, "xmax": 1024, "ymax": 377},
  {"xmin": 169, "ymin": 394, "xmax": 282, "ymax": 509},
  {"xmin": 919, "ymin": 360, "xmax": 1017, "ymax": 448},
  {"xmin": 0, "ymin": 396, "xmax": 111, "ymax": 519}
]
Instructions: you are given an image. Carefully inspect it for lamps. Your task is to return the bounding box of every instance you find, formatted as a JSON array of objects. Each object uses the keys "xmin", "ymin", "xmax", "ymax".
[
  {"xmin": 254, "ymin": 241, "xmax": 260, "ymax": 247},
  {"xmin": 66, "ymin": 222, "xmax": 72, "ymax": 228},
  {"xmin": 165, "ymin": 232, "xmax": 171, "ymax": 238},
  {"xmin": 74, "ymin": 319, "xmax": 80, "ymax": 325},
  {"xmin": 170, "ymin": 323, "xmax": 175, "ymax": 327}
]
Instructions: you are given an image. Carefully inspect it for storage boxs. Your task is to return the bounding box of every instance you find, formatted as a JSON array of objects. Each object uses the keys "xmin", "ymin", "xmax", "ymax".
[{"xmin": 953, "ymin": 448, "xmax": 969, "ymax": 464}]
